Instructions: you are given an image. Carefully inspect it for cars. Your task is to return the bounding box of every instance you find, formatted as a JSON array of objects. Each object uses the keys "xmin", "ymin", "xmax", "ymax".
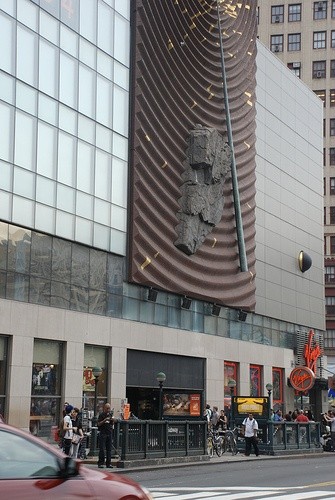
[{"xmin": 0, "ymin": 422, "xmax": 155, "ymax": 500}]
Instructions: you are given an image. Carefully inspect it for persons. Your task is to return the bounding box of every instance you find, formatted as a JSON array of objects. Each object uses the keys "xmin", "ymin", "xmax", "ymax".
[
  {"xmin": 271, "ymin": 409, "xmax": 313, "ymax": 445},
  {"xmin": 242, "ymin": 413, "xmax": 259, "ymax": 456},
  {"xmin": 315, "ymin": 410, "xmax": 335, "ymax": 452},
  {"xmin": 70, "ymin": 408, "xmax": 81, "ymax": 458},
  {"xmin": 203, "ymin": 404, "xmax": 227, "ymax": 430},
  {"xmin": 59, "ymin": 405, "xmax": 74, "ymax": 456},
  {"xmin": 97, "ymin": 403, "xmax": 115, "ymax": 469}
]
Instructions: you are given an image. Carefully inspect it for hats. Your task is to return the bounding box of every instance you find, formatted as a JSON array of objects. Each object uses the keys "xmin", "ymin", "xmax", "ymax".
[
  {"xmin": 299, "ymin": 409, "xmax": 304, "ymax": 413},
  {"xmin": 65, "ymin": 405, "xmax": 73, "ymax": 414}
]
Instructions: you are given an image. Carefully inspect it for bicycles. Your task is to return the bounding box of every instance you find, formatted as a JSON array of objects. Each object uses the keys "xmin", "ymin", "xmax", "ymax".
[{"xmin": 206, "ymin": 426, "xmax": 238, "ymax": 458}]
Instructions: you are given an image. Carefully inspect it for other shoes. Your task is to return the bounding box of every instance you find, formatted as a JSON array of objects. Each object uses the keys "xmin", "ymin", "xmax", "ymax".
[
  {"xmin": 106, "ymin": 464, "xmax": 114, "ymax": 468},
  {"xmin": 98, "ymin": 464, "xmax": 104, "ymax": 468}
]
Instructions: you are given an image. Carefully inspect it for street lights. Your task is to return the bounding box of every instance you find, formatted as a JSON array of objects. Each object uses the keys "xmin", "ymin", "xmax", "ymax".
[
  {"xmin": 265, "ymin": 383, "xmax": 274, "ymax": 454},
  {"xmin": 155, "ymin": 372, "xmax": 167, "ymax": 420},
  {"xmin": 228, "ymin": 378, "xmax": 237, "ymax": 425},
  {"xmin": 90, "ymin": 366, "xmax": 103, "ymax": 456}
]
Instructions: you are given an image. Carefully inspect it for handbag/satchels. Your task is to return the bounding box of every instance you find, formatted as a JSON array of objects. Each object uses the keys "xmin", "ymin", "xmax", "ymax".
[{"xmin": 71, "ymin": 433, "xmax": 82, "ymax": 445}]
